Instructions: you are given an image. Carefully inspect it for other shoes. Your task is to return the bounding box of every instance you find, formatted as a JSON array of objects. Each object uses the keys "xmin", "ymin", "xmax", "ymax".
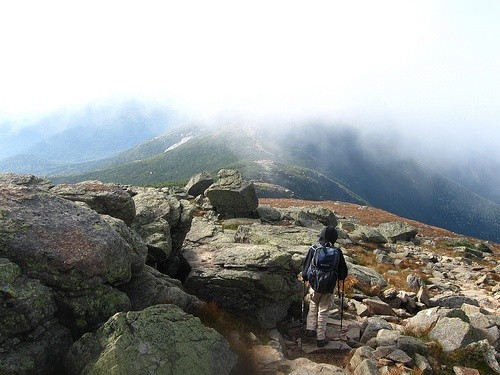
[
  {"xmin": 317, "ymin": 340, "xmax": 329, "ymax": 347},
  {"xmin": 303, "ymin": 330, "xmax": 315, "ymax": 339}
]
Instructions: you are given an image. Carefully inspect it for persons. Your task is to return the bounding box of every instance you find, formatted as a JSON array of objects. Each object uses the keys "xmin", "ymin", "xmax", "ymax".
[{"xmin": 296, "ymin": 226, "xmax": 349, "ymax": 348}]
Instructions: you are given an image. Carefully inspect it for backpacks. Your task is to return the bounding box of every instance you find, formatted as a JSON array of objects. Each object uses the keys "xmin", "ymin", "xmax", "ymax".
[{"xmin": 308, "ymin": 242, "xmax": 341, "ymax": 295}]
